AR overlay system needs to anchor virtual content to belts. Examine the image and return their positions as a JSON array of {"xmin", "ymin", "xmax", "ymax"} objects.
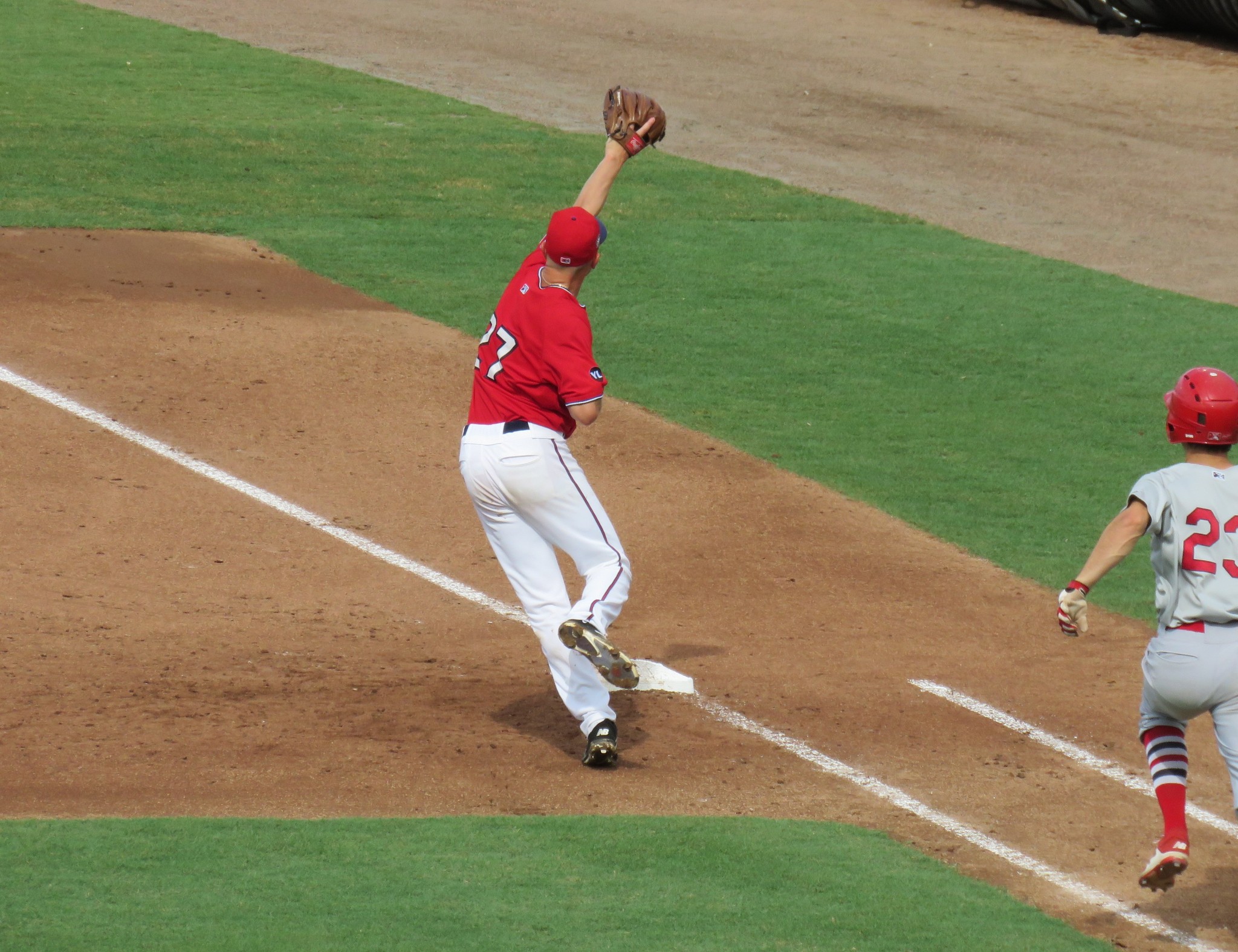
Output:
[
  {"xmin": 462, "ymin": 419, "xmax": 530, "ymax": 436},
  {"xmin": 1165, "ymin": 620, "xmax": 1205, "ymax": 633}
]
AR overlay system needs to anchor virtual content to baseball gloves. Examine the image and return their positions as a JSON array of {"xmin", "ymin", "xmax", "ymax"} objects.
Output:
[{"xmin": 601, "ymin": 83, "xmax": 669, "ymax": 159}]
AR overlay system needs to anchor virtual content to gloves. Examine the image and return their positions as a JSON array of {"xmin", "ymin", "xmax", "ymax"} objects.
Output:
[{"xmin": 1058, "ymin": 579, "xmax": 1091, "ymax": 639}]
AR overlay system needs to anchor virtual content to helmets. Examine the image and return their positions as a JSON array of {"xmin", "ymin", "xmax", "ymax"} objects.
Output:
[{"xmin": 1166, "ymin": 366, "xmax": 1238, "ymax": 445}]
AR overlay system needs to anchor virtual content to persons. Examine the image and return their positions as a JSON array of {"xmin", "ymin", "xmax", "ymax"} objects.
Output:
[
  {"xmin": 458, "ymin": 85, "xmax": 666, "ymax": 769},
  {"xmin": 1057, "ymin": 366, "xmax": 1238, "ymax": 894}
]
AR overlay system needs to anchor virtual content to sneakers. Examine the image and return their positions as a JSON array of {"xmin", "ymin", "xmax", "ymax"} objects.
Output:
[
  {"xmin": 558, "ymin": 617, "xmax": 639, "ymax": 689},
  {"xmin": 1138, "ymin": 833, "xmax": 1190, "ymax": 892},
  {"xmin": 580, "ymin": 718, "xmax": 618, "ymax": 768}
]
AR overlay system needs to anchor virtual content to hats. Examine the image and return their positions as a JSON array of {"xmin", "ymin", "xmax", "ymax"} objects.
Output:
[{"xmin": 547, "ymin": 206, "xmax": 607, "ymax": 267}]
